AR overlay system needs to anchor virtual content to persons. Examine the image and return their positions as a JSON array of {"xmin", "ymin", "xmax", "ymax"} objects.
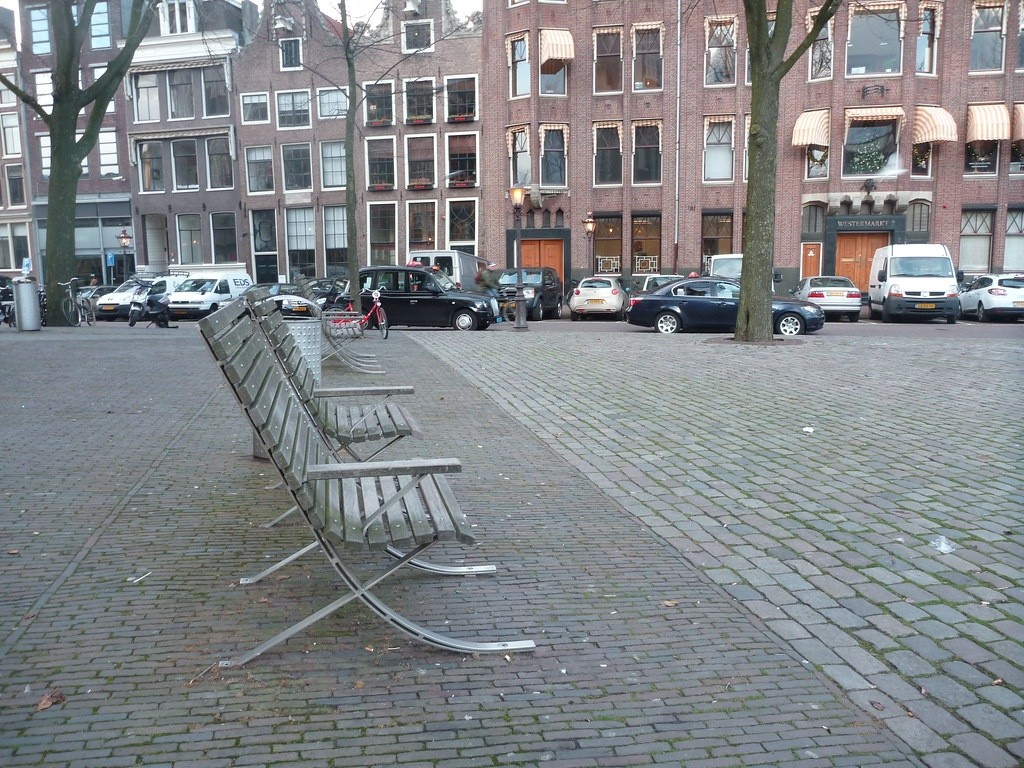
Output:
[{"xmin": 477, "ymin": 262, "xmax": 503, "ymax": 324}]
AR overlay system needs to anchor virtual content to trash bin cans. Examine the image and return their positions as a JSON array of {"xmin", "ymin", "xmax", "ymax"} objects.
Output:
[{"xmin": 12, "ymin": 275, "xmax": 43, "ymax": 330}]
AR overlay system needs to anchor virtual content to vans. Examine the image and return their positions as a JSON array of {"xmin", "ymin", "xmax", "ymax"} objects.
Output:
[
  {"xmin": 702, "ymin": 254, "xmax": 782, "ymax": 295},
  {"xmin": 867, "ymin": 244, "xmax": 963, "ymax": 324},
  {"xmin": 166, "ymin": 273, "xmax": 254, "ymax": 322},
  {"xmin": 407, "ymin": 250, "xmax": 494, "ymax": 293}
]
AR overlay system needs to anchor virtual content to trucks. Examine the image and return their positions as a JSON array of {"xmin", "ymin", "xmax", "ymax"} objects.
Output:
[{"xmin": 96, "ymin": 264, "xmax": 246, "ymax": 322}]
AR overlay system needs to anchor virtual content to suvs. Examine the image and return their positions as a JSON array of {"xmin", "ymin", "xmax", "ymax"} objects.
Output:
[{"xmin": 494, "ymin": 267, "xmax": 563, "ymax": 322}]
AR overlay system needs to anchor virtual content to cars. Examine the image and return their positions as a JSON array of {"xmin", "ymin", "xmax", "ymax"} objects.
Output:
[
  {"xmin": 217, "ymin": 279, "xmax": 342, "ymax": 316},
  {"xmin": 789, "ymin": 276, "xmax": 862, "ymax": 323},
  {"xmin": 335, "ymin": 262, "xmax": 492, "ymax": 333},
  {"xmin": 568, "ymin": 271, "xmax": 825, "ymax": 336},
  {"xmin": 957, "ymin": 274, "xmax": 1024, "ymax": 323},
  {"xmin": 78, "ymin": 285, "xmax": 118, "ymax": 314}
]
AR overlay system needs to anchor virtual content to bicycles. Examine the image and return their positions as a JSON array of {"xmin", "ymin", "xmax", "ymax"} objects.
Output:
[
  {"xmin": 0, "ymin": 286, "xmax": 47, "ymax": 328},
  {"xmin": 322, "ymin": 288, "xmax": 389, "ymax": 340},
  {"xmin": 57, "ymin": 278, "xmax": 96, "ymax": 327},
  {"xmin": 492, "ymin": 286, "xmax": 527, "ymax": 323}
]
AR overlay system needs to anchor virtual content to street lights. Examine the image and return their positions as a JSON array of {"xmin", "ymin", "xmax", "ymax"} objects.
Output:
[
  {"xmin": 581, "ymin": 212, "xmax": 597, "ymax": 277},
  {"xmin": 507, "ymin": 182, "xmax": 530, "ymax": 330},
  {"xmin": 115, "ymin": 229, "xmax": 133, "ymax": 282}
]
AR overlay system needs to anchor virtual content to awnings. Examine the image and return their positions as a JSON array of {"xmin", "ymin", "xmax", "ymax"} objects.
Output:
[
  {"xmin": 1013, "ymin": 104, "xmax": 1024, "ymax": 142},
  {"xmin": 592, "ymin": 25, "xmax": 624, "ymax": 61},
  {"xmin": 128, "ymin": 127, "xmax": 237, "ymax": 165},
  {"xmin": 805, "ymin": 6, "xmax": 835, "ymax": 41},
  {"xmin": 503, "ymin": 32, "xmax": 530, "ymax": 68},
  {"xmin": 540, "ymin": 29, "xmax": 575, "ymax": 67},
  {"xmin": 592, "ymin": 118, "xmax": 662, "ymax": 155},
  {"xmin": 701, "ymin": 114, "xmax": 753, "ymax": 150},
  {"xmin": 847, "ymin": 1, "xmax": 908, "ymax": 38},
  {"xmin": 918, "ymin": 1, "xmax": 943, "ymax": 39},
  {"xmin": 703, "ymin": 14, "xmax": 739, "ymax": 53},
  {"xmin": 913, "ymin": 104, "xmax": 959, "ymax": 145},
  {"xmin": 629, "ymin": 20, "xmax": 664, "ymax": 59},
  {"xmin": 505, "ymin": 124, "xmax": 571, "ymax": 158},
  {"xmin": 970, "ymin": 0, "xmax": 1011, "ymax": 39},
  {"xmin": 966, "ymin": 104, "xmax": 1011, "ymax": 144},
  {"xmin": 845, "ymin": 106, "xmax": 906, "ymax": 143},
  {"xmin": 125, "ymin": 57, "xmax": 231, "ymax": 98},
  {"xmin": 791, "ymin": 110, "xmax": 829, "ymax": 147}
]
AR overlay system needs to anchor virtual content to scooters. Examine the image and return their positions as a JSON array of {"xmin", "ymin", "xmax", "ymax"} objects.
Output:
[{"xmin": 128, "ymin": 275, "xmax": 170, "ymax": 328}]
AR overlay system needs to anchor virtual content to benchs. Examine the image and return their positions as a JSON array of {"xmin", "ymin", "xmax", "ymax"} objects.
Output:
[{"xmin": 198, "ymin": 279, "xmax": 537, "ymax": 668}]
